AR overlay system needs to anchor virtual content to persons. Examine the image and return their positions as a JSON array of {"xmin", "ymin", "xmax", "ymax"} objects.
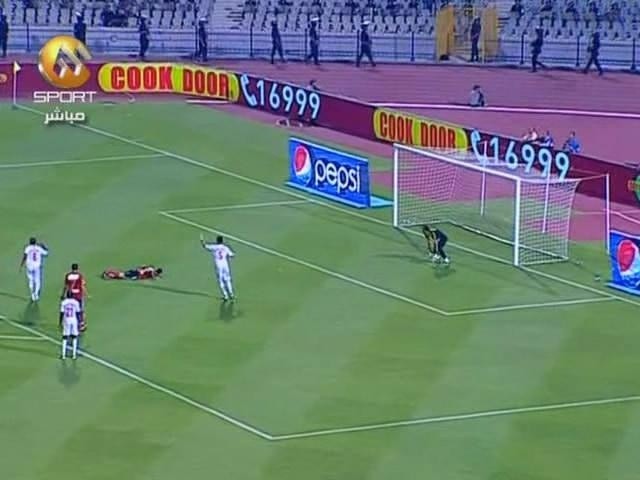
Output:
[
  {"xmin": 468, "ymin": 17, "xmax": 481, "ymax": 62},
  {"xmin": 270, "ymin": 21, "xmax": 287, "ymax": 64},
  {"xmin": 302, "ymin": 18, "xmax": 322, "ymax": 65},
  {"xmin": 580, "ymin": 32, "xmax": 604, "ymax": 76},
  {"xmin": 0, "ymin": 10, "xmax": 9, "ymax": 58},
  {"xmin": 528, "ymin": 28, "xmax": 547, "ymax": 73},
  {"xmin": 18, "ymin": 237, "xmax": 88, "ymax": 360},
  {"xmin": 196, "ymin": 18, "xmax": 210, "ymax": 63},
  {"xmin": 522, "ymin": 126, "xmax": 581, "ymax": 154},
  {"xmin": 72, "ymin": 11, "xmax": 87, "ymax": 58},
  {"xmin": 307, "ymin": 79, "xmax": 320, "ymax": 91},
  {"xmin": 138, "ymin": 16, "xmax": 150, "ymax": 61},
  {"xmin": 199, "ymin": 232, "xmax": 235, "ymax": 301},
  {"xmin": 422, "ymin": 223, "xmax": 449, "ymax": 264},
  {"xmin": 469, "ymin": 84, "xmax": 485, "ymax": 107},
  {"xmin": 509, "ymin": 1, "xmax": 623, "ymax": 14},
  {"xmin": 358, "ymin": 21, "xmax": 377, "ymax": 67},
  {"xmin": 102, "ymin": 263, "xmax": 163, "ymax": 280}
]
